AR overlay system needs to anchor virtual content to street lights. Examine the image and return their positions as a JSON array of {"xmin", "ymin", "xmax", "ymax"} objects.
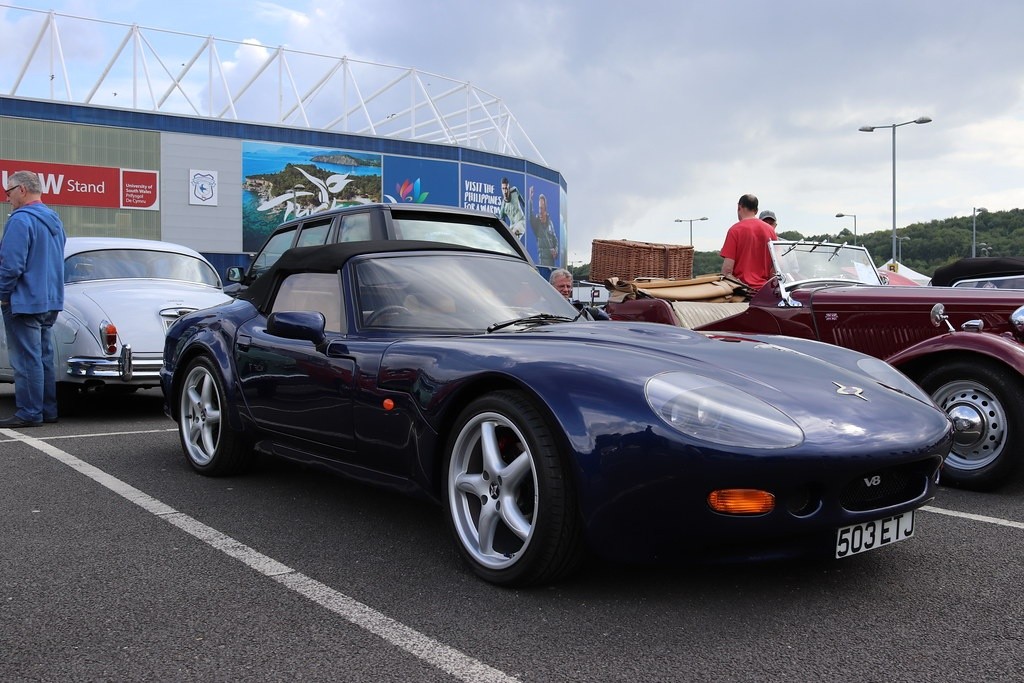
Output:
[
  {"xmin": 568, "ymin": 261, "xmax": 582, "ymax": 274},
  {"xmin": 858, "ymin": 116, "xmax": 932, "ymax": 265},
  {"xmin": 890, "ymin": 235, "xmax": 910, "ymax": 264},
  {"xmin": 835, "ymin": 213, "xmax": 856, "ymax": 245},
  {"xmin": 972, "ymin": 207, "xmax": 988, "ymax": 258},
  {"xmin": 675, "ymin": 217, "xmax": 708, "ymax": 245}
]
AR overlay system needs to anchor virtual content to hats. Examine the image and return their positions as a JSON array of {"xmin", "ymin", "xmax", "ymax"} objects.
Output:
[{"xmin": 759, "ymin": 210, "xmax": 777, "ymax": 222}]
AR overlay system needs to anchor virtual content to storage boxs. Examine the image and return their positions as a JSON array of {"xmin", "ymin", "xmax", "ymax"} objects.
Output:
[{"xmin": 573, "ymin": 286, "xmax": 609, "ymax": 303}]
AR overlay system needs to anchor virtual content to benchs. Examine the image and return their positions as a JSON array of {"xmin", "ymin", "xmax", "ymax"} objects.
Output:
[{"xmin": 671, "ymin": 301, "xmax": 749, "ymax": 329}]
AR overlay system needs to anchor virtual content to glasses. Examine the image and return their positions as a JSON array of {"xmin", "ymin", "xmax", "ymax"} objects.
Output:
[{"xmin": 5, "ymin": 184, "xmax": 21, "ymax": 198}]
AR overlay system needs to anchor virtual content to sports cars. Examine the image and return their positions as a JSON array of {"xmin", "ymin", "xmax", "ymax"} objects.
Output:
[
  {"xmin": 158, "ymin": 240, "xmax": 955, "ymax": 587},
  {"xmin": 607, "ymin": 239, "xmax": 1024, "ymax": 490},
  {"xmin": 932, "ymin": 258, "xmax": 1024, "ymax": 291}
]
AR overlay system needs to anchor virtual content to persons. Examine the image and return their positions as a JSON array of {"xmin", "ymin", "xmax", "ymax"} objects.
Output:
[
  {"xmin": 0, "ymin": 170, "xmax": 66, "ymax": 428},
  {"xmin": 550, "ymin": 269, "xmax": 573, "ymax": 300},
  {"xmin": 720, "ymin": 193, "xmax": 777, "ymax": 292},
  {"xmin": 528, "ymin": 187, "xmax": 559, "ymax": 267},
  {"xmin": 498, "ymin": 178, "xmax": 526, "ymax": 240},
  {"xmin": 759, "ymin": 210, "xmax": 799, "ymax": 274}
]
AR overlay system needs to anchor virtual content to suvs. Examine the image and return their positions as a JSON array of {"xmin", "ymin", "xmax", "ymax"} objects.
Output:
[{"xmin": 224, "ymin": 203, "xmax": 540, "ymax": 300}]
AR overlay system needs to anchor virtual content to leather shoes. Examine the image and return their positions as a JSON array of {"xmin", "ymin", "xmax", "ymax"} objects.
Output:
[
  {"xmin": 44, "ymin": 418, "xmax": 58, "ymax": 423},
  {"xmin": 0, "ymin": 414, "xmax": 43, "ymax": 428}
]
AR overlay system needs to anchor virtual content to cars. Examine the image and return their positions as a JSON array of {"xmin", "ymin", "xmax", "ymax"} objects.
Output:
[{"xmin": 0, "ymin": 237, "xmax": 234, "ymax": 413}]
